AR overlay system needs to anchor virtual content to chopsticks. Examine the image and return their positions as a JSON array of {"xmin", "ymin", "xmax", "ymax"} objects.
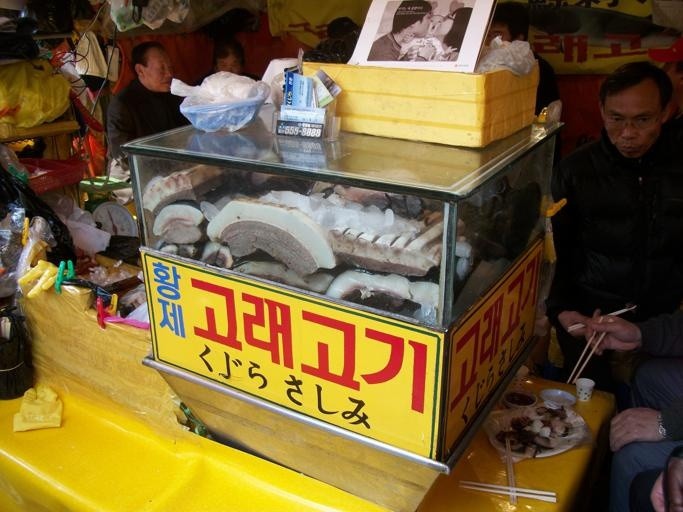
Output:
[
  {"xmin": 458, "ymin": 480, "xmax": 557, "ymax": 504},
  {"xmin": 567, "ymin": 306, "xmax": 637, "ymax": 332},
  {"xmin": 505, "ymin": 439, "xmax": 517, "ymax": 506},
  {"xmin": 567, "ymin": 316, "xmax": 614, "ymax": 384}
]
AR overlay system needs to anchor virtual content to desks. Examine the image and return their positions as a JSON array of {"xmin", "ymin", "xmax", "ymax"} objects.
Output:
[{"xmin": 0, "ymin": 256, "xmax": 614, "ymax": 512}]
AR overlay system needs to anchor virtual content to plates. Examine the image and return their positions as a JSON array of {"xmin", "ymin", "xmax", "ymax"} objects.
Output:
[
  {"xmin": 539, "ymin": 389, "xmax": 576, "ymax": 406},
  {"xmin": 503, "ymin": 390, "xmax": 537, "ymax": 408},
  {"xmin": 489, "ymin": 404, "xmax": 586, "ymax": 458}
]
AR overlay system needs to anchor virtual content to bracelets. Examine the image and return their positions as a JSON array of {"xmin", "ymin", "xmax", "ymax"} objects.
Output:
[{"xmin": 657, "ymin": 412, "xmax": 666, "ymax": 440}]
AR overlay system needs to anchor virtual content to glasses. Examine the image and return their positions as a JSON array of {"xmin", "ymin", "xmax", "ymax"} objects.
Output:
[{"xmin": 602, "ymin": 108, "xmax": 664, "ymax": 130}]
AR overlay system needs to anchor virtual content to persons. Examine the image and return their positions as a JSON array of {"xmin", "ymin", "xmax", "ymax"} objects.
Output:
[
  {"xmin": 552, "ymin": 61, "xmax": 681, "ymax": 411},
  {"xmin": 432, "ymin": 7, "xmax": 473, "ymax": 62},
  {"xmin": 103, "ymin": 41, "xmax": 192, "ymax": 201},
  {"xmin": 399, "ymin": 15, "xmax": 457, "ymax": 62},
  {"xmin": 367, "ymin": 0, "xmax": 433, "ymax": 61},
  {"xmin": 187, "ymin": 38, "xmax": 259, "ymax": 89},
  {"xmin": 586, "ymin": 304, "xmax": 683, "ymax": 511},
  {"xmin": 629, "ymin": 444, "xmax": 682, "ymax": 512},
  {"xmin": 300, "ymin": 16, "xmax": 362, "ymax": 64}
]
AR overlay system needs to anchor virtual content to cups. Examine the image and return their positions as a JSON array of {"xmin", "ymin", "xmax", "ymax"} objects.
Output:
[{"xmin": 575, "ymin": 378, "xmax": 595, "ymax": 401}]
